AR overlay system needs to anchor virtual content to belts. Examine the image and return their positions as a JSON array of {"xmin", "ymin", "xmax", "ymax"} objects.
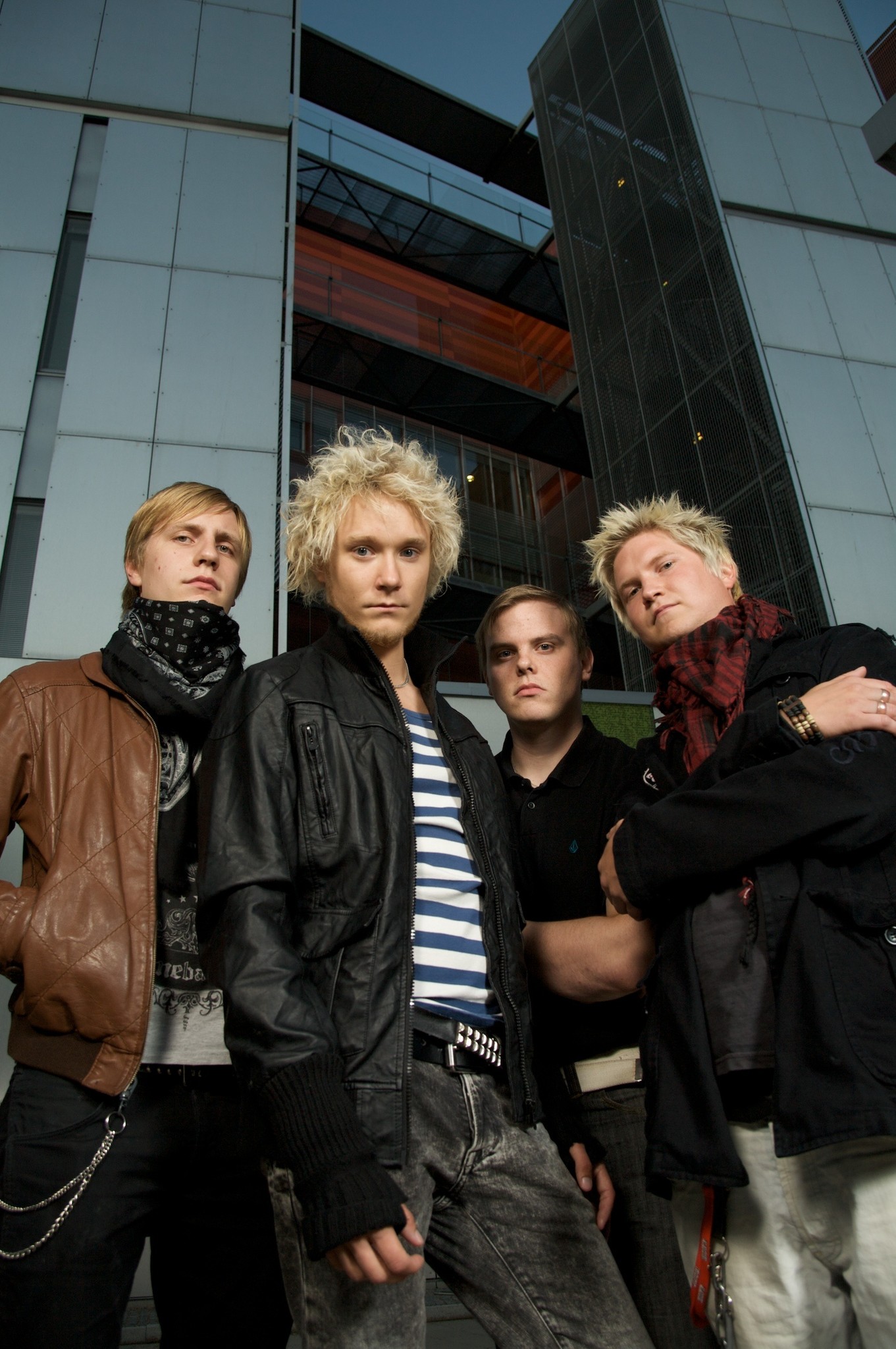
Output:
[
  {"xmin": 575, "ymin": 1048, "xmax": 644, "ymax": 1093},
  {"xmin": 407, "ymin": 1033, "xmax": 486, "ymax": 1074},
  {"xmin": 405, "ymin": 1014, "xmax": 503, "ymax": 1068}
]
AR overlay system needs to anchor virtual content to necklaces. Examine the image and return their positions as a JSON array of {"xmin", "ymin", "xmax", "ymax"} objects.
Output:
[{"xmin": 393, "ymin": 658, "xmax": 410, "ymax": 688}]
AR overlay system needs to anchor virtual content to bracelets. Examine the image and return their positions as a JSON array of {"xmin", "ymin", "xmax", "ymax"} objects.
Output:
[{"xmin": 776, "ymin": 694, "xmax": 826, "ymax": 746}]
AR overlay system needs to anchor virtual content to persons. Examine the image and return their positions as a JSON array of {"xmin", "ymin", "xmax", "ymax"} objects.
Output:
[
  {"xmin": 0, "ymin": 483, "xmax": 294, "ymax": 1349},
  {"xmin": 196, "ymin": 423, "xmax": 656, "ymax": 1349},
  {"xmin": 584, "ymin": 491, "xmax": 896, "ymax": 1349},
  {"xmin": 476, "ymin": 585, "xmax": 724, "ymax": 1349}
]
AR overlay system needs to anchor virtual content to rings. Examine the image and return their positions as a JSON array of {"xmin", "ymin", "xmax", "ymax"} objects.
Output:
[
  {"xmin": 880, "ymin": 689, "xmax": 890, "ymax": 703},
  {"xmin": 875, "ymin": 702, "xmax": 887, "ymax": 716}
]
